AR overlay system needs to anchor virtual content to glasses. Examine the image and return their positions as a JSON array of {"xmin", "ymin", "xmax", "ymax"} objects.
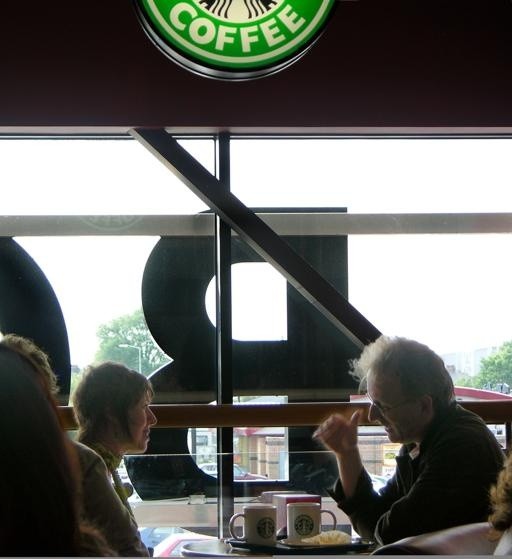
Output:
[{"xmin": 366, "ymin": 392, "xmax": 420, "ymax": 415}]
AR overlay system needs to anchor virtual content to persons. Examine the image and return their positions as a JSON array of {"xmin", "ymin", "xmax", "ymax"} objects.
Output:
[
  {"xmin": 68, "ymin": 357, "xmax": 159, "ymax": 556},
  {"xmin": 1, "ymin": 331, "xmax": 123, "ymax": 557},
  {"xmin": 310, "ymin": 332, "xmax": 511, "ymax": 546},
  {"xmin": 481, "ymin": 439, "xmax": 512, "ymax": 558}
]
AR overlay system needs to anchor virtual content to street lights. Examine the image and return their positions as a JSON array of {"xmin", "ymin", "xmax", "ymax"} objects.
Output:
[{"xmin": 118, "ymin": 343, "xmax": 142, "ymax": 374}]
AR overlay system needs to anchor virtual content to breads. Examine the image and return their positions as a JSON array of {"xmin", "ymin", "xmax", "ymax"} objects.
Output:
[{"xmin": 311, "ymin": 531, "xmax": 350, "ymax": 544}]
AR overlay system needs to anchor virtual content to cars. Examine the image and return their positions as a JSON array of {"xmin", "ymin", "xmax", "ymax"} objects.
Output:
[{"xmin": 200, "ymin": 462, "xmax": 269, "ymax": 480}]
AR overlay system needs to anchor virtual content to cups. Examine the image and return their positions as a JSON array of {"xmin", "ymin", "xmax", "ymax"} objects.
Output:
[
  {"xmin": 286, "ymin": 503, "xmax": 338, "ymax": 538},
  {"xmin": 228, "ymin": 507, "xmax": 279, "ymax": 547}
]
